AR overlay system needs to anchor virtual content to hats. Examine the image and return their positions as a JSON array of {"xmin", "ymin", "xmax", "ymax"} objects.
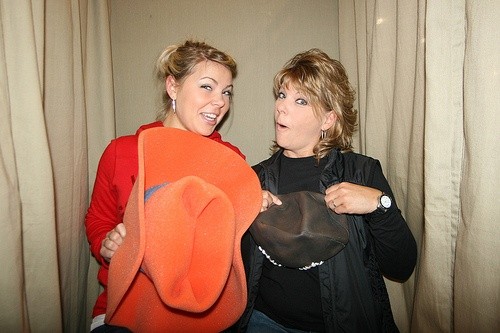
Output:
[
  {"xmin": 105, "ymin": 127, "xmax": 262, "ymax": 333},
  {"xmin": 250, "ymin": 191, "xmax": 350, "ymax": 269}
]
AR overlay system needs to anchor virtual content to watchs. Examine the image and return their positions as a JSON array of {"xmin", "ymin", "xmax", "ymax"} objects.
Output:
[{"xmin": 373, "ymin": 185, "xmax": 394, "ymax": 217}]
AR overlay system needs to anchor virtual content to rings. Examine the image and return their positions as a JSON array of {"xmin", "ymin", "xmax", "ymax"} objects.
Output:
[{"xmin": 332, "ymin": 199, "xmax": 337, "ymax": 208}]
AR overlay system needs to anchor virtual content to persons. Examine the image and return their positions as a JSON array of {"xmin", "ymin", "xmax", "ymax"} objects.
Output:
[
  {"xmin": 85, "ymin": 42, "xmax": 262, "ymax": 333},
  {"xmin": 237, "ymin": 49, "xmax": 418, "ymax": 333}
]
